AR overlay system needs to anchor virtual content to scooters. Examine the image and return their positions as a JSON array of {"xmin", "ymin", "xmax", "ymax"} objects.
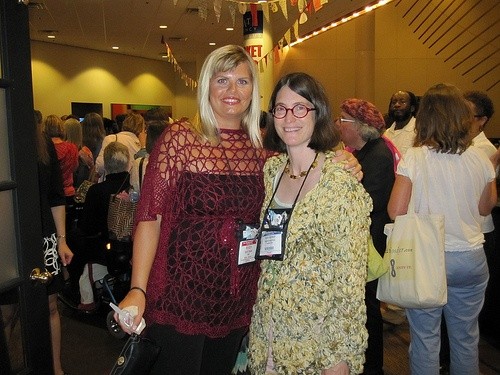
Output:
[{"xmin": 58, "ymin": 240, "xmax": 134, "ymax": 340}]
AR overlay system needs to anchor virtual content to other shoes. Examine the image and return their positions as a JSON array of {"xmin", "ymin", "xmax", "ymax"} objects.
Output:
[{"xmin": 79, "ymin": 301, "xmax": 96, "ymax": 311}]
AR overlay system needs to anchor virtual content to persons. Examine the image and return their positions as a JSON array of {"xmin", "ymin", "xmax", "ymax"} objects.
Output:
[
  {"xmin": 45, "ymin": 116, "xmax": 78, "ymax": 288},
  {"xmin": 335, "ymin": 98, "xmax": 395, "ymax": 374},
  {"xmin": 61, "ymin": 142, "xmax": 130, "ymax": 310},
  {"xmin": 247, "ymin": 72, "xmax": 374, "ymax": 374},
  {"xmin": 112, "ymin": 44, "xmax": 364, "ymax": 375},
  {"xmin": 37, "ymin": 127, "xmax": 74, "ymax": 375},
  {"xmin": 462, "ymin": 89, "xmax": 499, "ymax": 346},
  {"xmin": 129, "ymin": 121, "xmax": 173, "ymax": 199},
  {"xmin": 387, "ymin": 83, "xmax": 497, "ymax": 374},
  {"xmin": 380, "ymin": 88, "xmax": 416, "ymax": 162},
  {"xmin": 62, "ymin": 118, "xmax": 94, "ymax": 200},
  {"xmin": 33, "ymin": 107, "xmax": 179, "ymax": 182}
]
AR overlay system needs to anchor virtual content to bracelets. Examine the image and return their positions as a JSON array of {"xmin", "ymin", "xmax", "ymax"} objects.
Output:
[
  {"xmin": 55, "ymin": 233, "xmax": 66, "ymax": 238},
  {"xmin": 129, "ymin": 286, "xmax": 146, "ymax": 299}
]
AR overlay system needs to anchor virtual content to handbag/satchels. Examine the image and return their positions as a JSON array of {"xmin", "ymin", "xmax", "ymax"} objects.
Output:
[
  {"xmin": 109, "ymin": 287, "xmax": 152, "ymax": 375},
  {"xmin": 75, "ymin": 165, "xmax": 99, "ymax": 204},
  {"xmin": 107, "ymin": 163, "xmax": 138, "ymax": 242},
  {"xmin": 377, "ymin": 149, "xmax": 447, "ymax": 310}
]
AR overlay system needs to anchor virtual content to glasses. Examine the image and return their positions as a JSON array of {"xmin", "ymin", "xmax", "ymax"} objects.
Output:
[
  {"xmin": 338, "ymin": 116, "xmax": 356, "ymax": 124},
  {"xmin": 272, "ymin": 104, "xmax": 316, "ymax": 118}
]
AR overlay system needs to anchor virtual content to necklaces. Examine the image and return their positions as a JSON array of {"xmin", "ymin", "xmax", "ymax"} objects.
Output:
[{"xmin": 284, "ymin": 151, "xmax": 321, "ymax": 180}]
